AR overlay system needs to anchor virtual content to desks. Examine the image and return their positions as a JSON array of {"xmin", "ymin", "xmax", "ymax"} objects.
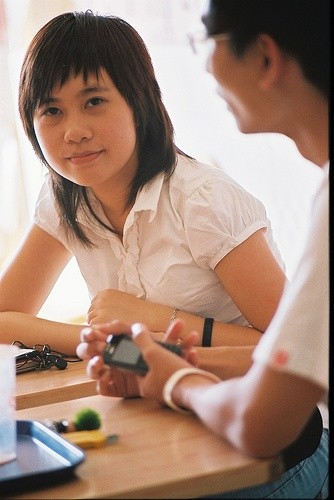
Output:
[{"xmin": 0, "ymin": 354, "xmax": 324, "ymax": 500}]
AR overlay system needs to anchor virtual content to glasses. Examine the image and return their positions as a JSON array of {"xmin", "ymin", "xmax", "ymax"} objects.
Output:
[{"xmin": 186, "ymin": 28, "xmax": 231, "ymax": 54}]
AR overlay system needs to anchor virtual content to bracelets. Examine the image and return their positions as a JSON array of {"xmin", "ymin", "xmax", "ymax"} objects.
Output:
[
  {"xmin": 167, "ymin": 306, "xmax": 182, "ymax": 345},
  {"xmin": 201, "ymin": 318, "xmax": 214, "ymax": 347},
  {"xmin": 163, "ymin": 366, "xmax": 223, "ymax": 415}
]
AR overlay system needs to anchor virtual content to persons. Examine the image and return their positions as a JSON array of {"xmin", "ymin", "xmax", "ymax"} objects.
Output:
[
  {"xmin": 76, "ymin": 0, "xmax": 330, "ymax": 459},
  {"xmin": 0, "ymin": 9, "xmax": 328, "ymax": 499}
]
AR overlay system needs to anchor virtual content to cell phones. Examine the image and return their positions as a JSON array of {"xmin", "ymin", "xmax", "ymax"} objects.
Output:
[{"xmin": 103, "ymin": 334, "xmax": 182, "ymax": 374}]
